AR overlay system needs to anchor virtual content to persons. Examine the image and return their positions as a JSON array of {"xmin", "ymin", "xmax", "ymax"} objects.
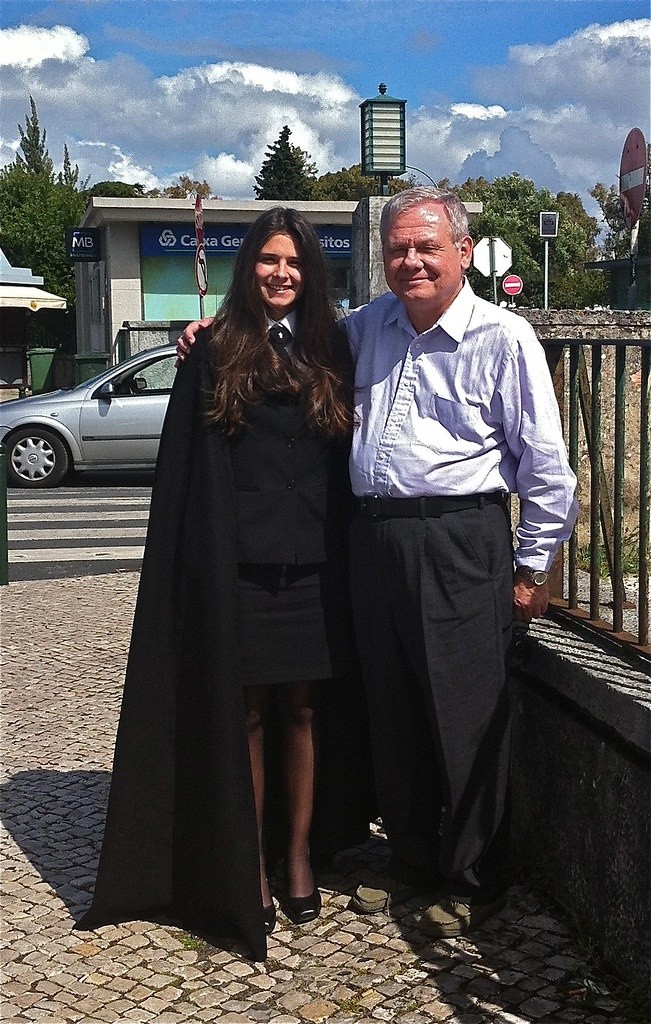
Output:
[
  {"xmin": 72, "ymin": 206, "xmax": 355, "ymax": 962},
  {"xmin": 174, "ymin": 186, "xmax": 579, "ymax": 939}
]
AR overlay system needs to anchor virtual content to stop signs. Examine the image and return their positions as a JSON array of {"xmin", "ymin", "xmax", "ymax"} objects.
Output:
[
  {"xmin": 619, "ymin": 126, "xmax": 647, "ymax": 232},
  {"xmin": 502, "ymin": 275, "xmax": 523, "ymax": 295}
]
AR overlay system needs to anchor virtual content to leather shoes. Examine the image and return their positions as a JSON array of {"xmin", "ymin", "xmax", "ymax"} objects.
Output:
[
  {"xmin": 284, "ymin": 887, "xmax": 321, "ymax": 924},
  {"xmin": 263, "ymin": 904, "xmax": 276, "ymax": 933}
]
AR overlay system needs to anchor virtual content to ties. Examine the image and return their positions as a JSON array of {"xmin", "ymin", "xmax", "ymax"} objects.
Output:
[{"xmin": 267, "ymin": 327, "xmax": 293, "ymax": 383}]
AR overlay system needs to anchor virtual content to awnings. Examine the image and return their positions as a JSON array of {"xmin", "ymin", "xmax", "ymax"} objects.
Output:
[{"xmin": 0, "ymin": 286, "xmax": 67, "ymax": 311}]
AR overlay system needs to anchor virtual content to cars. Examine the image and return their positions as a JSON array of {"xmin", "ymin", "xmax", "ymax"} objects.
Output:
[{"xmin": 0, "ymin": 344, "xmax": 180, "ymax": 486}]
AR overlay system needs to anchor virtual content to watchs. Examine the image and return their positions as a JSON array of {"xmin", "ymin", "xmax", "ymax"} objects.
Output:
[{"xmin": 515, "ymin": 564, "xmax": 549, "ymax": 586}]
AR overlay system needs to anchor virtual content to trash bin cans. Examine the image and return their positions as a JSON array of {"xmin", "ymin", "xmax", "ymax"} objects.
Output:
[
  {"xmin": 74, "ymin": 351, "xmax": 111, "ymax": 385},
  {"xmin": 26, "ymin": 348, "xmax": 59, "ymax": 395}
]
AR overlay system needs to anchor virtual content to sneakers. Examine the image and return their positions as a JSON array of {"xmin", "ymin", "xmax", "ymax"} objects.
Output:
[
  {"xmin": 354, "ymin": 843, "xmax": 440, "ymax": 913},
  {"xmin": 418, "ymin": 897, "xmax": 508, "ymax": 937}
]
